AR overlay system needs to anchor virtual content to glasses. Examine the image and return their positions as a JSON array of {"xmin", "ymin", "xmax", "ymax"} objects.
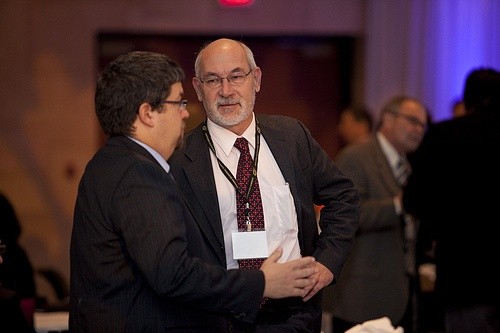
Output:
[
  {"xmin": 199, "ymin": 69, "xmax": 252, "ymax": 88},
  {"xmin": 160, "ymin": 100, "xmax": 188, "ymax": 111},
  {"xmin": 393, "ymin": 112, "xmax": 428, "ymax": 133}
]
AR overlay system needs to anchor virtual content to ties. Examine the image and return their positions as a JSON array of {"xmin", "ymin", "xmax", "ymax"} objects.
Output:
[
  {"xmin": 233, "ymin": 137, "xmax": 268, "ymax": 313},
  {"xmin": 394, "ymin": 158, "xmax": 418, "ymax": 280}
]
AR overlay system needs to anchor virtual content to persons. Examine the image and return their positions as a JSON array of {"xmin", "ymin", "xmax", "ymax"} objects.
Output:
[
  {"xmin": 69, "ymin": 51, "xmax": 319, "ymax": 333},
  {"xmin": 334, "ymin": 102, "xmax": 373, "ymax": 145},
  {"xmin": 167, "ymin": 38, "xmax": 362, "ymax": 333},
  {"xmin": 401, "ymin": 66, "xmax": 500, "ymax": 333},
  {"xmin": 319, "ymin": 96, "xmax": 426, "ymax": 333}
]
